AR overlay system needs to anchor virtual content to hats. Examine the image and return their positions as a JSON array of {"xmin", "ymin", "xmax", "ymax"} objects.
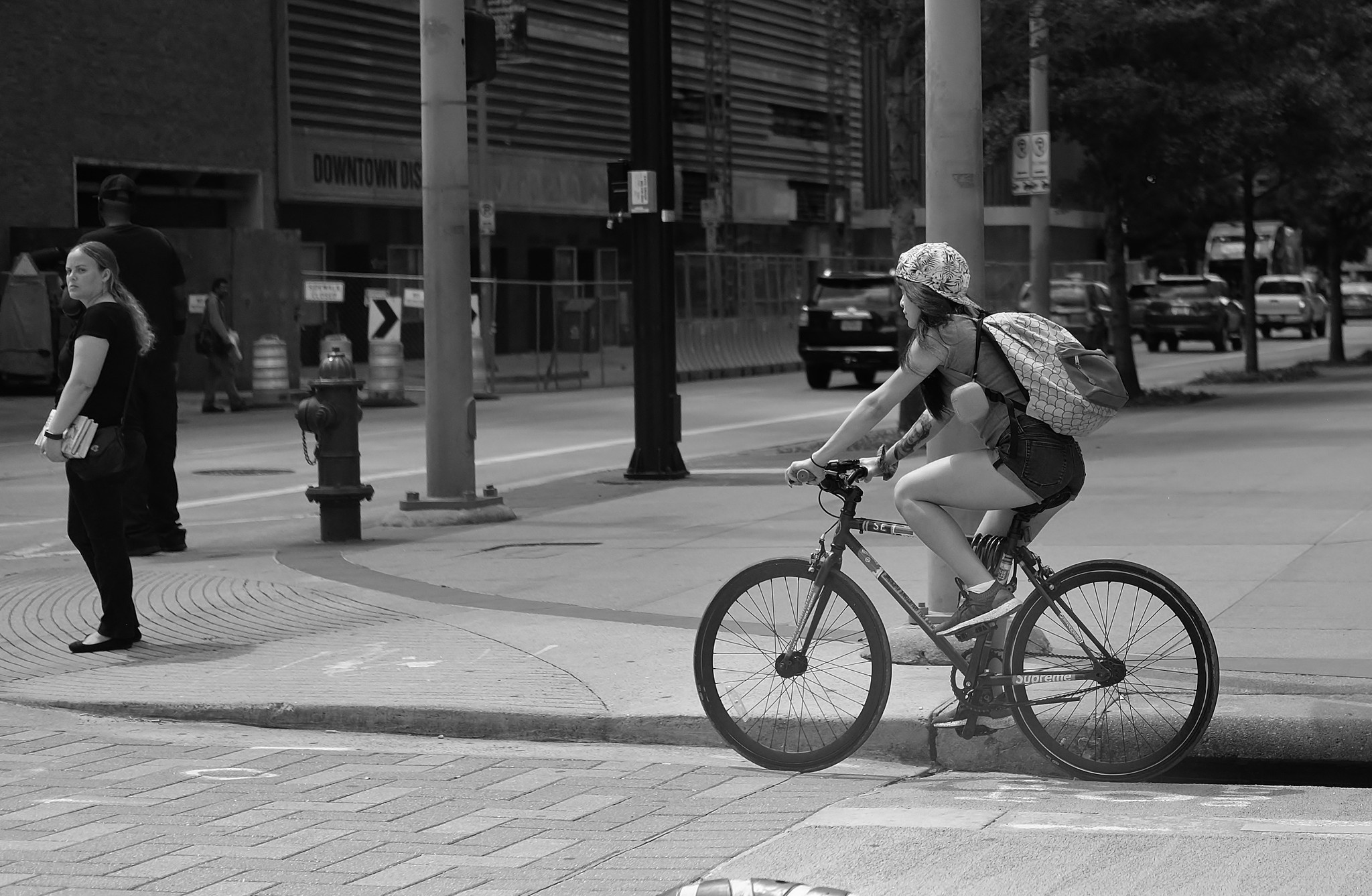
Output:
[
  {"xmin": 94, "ymin": 173, "xmax": 139, "ymax": 204},
  {"xmin": 895, "ymin": 241, "xmax": 979, "ymax": 308}
]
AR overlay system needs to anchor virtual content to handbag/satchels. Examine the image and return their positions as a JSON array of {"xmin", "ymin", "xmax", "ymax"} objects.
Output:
[
  {"xmin": 196, "ymin": 331, "xmax": 210, "ymax": 355},
  {"xmin": 72, "ymin": 425, "xmax": 126, "ymax": 482}
]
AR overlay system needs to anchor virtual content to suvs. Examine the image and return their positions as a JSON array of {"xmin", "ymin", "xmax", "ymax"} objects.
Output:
[{"xmin": 796, "ymin": 269, "xmax": 915, "ymax": 390}]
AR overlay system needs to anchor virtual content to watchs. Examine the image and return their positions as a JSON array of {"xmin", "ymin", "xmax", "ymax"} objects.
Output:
[{"xmin": 44, "ymin": 430, "xmax": 63, "ymax": 440}]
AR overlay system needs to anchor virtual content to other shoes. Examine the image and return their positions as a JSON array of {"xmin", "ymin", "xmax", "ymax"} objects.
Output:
[
  {"xmin": 203, "ymin": 404, "xmax": 225, "ymax": 414},
  {"xmin": 231, "ymin": 405, "xmax": 252, "ymax": 413},
  {"xmin": 126, "ymin": 542, "xmax": 160, "ymax": 557},
  {"xmin": 69, "ymin": 625, "xmax": 141, "ymax": 652},
  {"xmin": 160, "ymin": 533, "xmax": 187, "ymax": 552}
]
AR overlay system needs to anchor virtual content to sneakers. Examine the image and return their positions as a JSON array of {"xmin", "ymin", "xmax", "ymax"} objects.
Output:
[
  {"xmin": 941, "ymin": 577, "xmax": 1025, "ymax": 639},
  {"xmin": 933, "ymin": 690, "xmax": 1015, "ymax": 730}
]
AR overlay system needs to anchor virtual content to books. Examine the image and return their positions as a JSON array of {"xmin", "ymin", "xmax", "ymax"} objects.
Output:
[{"xmin": 34, "ymin": 409, "xmax": 99, "ymax": 459}]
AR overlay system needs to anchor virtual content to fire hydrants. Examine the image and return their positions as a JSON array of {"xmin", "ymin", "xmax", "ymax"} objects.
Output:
[{"xmin": 294, "ymin": 346, "xmax": 375, "ymax": 542}]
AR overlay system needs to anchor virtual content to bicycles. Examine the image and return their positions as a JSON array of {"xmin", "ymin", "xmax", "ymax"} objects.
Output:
[{"xmin": 692, "ymin": 463, "xmax": 1221, "ymax": 785}]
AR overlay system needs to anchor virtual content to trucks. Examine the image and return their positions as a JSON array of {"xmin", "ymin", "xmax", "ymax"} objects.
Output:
[{"xmin": 1202, "ymin": 221, "xmax": 1304, "ymax": 302}]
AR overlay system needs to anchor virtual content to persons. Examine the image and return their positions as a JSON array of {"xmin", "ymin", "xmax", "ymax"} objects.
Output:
[
  {"xmin": 41, "ymin": 241, "xmax": 154, "ymax": 654},
  {"xmin": 785, "ymin": 242, "xmax": 1086, "ymax": 729},
  {"xmin": 58, "ymin": 174, "xmax": 188, "ymax": 558},
  {"xmin": 200, "ymin": 278, "xmax": 248, "ymax": 413}
]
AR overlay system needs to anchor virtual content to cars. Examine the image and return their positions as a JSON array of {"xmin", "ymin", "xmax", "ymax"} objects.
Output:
[
  {"xmin": 1340, "ymin": 280, "xmax": 1372, "ymax": 316},
  {"xmin": 1016, "ymin": 279, "xmax": 1114, "ymax": 351},
  {"xmin": 1126, "ymin": 280, "xmax": 1156, "ymax": 328},
  {"xmin": 1253, "ymin": 274, "xmax": 1328, "ymax": 340},
  {"xmin": 1142, "ymin": 272, "xmax": 1245, "ymax": 353}
]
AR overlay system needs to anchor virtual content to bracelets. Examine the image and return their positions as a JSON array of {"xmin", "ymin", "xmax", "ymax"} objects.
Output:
[
  {"xmin": 876, "ymin": 444, "xmax": 899, "ymax": 481},
  {"xmin": 810, "ymin": 456, "xmax": 825, "ymax": 468}
]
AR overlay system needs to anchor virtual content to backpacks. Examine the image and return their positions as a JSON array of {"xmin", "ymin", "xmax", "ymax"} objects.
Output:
[{"xmin": 945, "ymin": 312, "xmax": 1129, "ymax": 436}]
